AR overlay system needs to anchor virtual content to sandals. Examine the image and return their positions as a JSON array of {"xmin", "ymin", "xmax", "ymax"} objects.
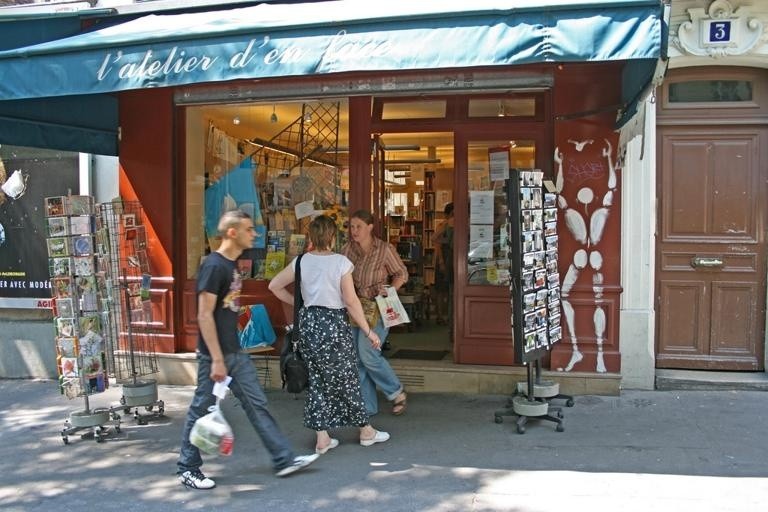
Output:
[{"xmin": 391, "ymin": 391, "xmax": 408, "ymax": 416}]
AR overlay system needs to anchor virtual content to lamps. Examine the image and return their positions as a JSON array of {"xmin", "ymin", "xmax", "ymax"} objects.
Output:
[
  {"xmin": 305, "ymin": 103, "xmax": 312, "ymax": 123},
  {"xmin": 496, "ymin": 100, "xmax": 504, "ymax": 117},
  {"xmin": 233, "ymin": 106, "xmax": 240, "ymax": 126},
  {"xmin": 510, "ymin": 140, "xmax": 518, "ymax": 149},
  {"xmin": 270, "ymin": 104, "xmax": 278, "ymax": 123}
]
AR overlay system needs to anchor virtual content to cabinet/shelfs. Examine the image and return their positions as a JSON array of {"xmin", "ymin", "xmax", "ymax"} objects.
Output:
[{"xmin": 383, "ymin": 164, "xmax": 453, "ymax": 334}]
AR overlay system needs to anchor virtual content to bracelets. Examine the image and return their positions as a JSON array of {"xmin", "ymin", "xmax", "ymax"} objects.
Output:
[{"xmin": 365, "ymin": 328, "xmax": 370, "ymax": 338}]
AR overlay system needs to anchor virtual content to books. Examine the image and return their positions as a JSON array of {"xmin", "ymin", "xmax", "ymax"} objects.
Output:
[
  {"xmin": 508, "ymin": 167, "xmax": 563, "ymax": 352},
  {"xmin": 45, "ymin": 196, "xmax": 152, "ymax": 400},
  {"xmin": 234, "ymin": 230, "xmax": 306, "ymax": 282}
]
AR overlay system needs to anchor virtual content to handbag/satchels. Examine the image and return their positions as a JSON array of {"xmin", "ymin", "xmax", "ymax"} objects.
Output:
[
  {"xmin": 346, "ymin": 298, "xmax": 380, "ymax": 328},
  {"xmin": 279, "ymin": 333, "xmax": 308, "ymax": 394},
  {"xmin": 375, "ymin": 285, "xmax": 411, "ymax": 330}
]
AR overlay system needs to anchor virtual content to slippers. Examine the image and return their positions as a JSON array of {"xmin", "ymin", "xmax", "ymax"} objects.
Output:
[
  {"xmin": 359, "ymin": 430, "xmax": 391, "ymax": 447},
  {"xmin": 316, "ymin": 438, "xmax": 339, "ymax": 455}
]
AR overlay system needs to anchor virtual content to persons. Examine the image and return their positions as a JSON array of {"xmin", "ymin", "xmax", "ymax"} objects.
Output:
[
  {"xmin": 177, "ymin": 209, "xmax": 319, "ymax": 488},
  {"xmin": 268, "ymin": 215, "xmax": 390, "ymax": 456},
  {"xmin": 340, "ymin": 210, "xmax": 409, "ymax": 417},
  {"xmin": 432, "ymin": 202, "xmax": 454, "ymax": 324}
]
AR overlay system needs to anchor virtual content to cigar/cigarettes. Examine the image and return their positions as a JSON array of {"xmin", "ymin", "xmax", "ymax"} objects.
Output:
[{"xmin": 378, "ymin": 346, "xmax": 382, "ymax": 352}]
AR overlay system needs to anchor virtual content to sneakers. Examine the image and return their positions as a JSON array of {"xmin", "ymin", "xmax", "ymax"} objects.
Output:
[
  {"xmin": 274, "ymin": 453, "xmax": 321, "ymax": 478},
  {"xmin": 177, "ymin": 469, "xmax": 217, "ymax": 489}
]
